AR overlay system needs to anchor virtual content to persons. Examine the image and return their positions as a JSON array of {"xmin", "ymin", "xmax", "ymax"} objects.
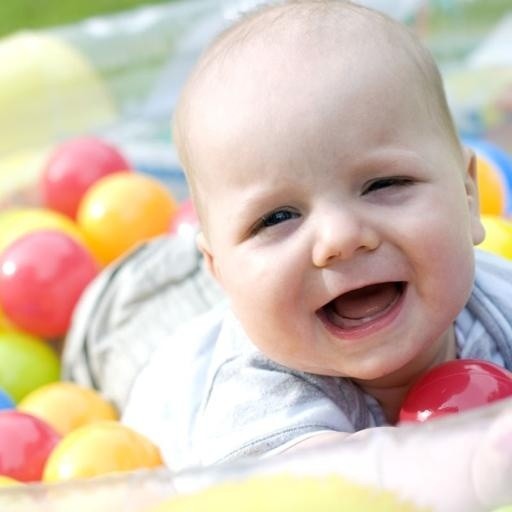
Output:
[{"xmin": 59, "ymin": 0, "xmax": 512, "ymax": 512}]
